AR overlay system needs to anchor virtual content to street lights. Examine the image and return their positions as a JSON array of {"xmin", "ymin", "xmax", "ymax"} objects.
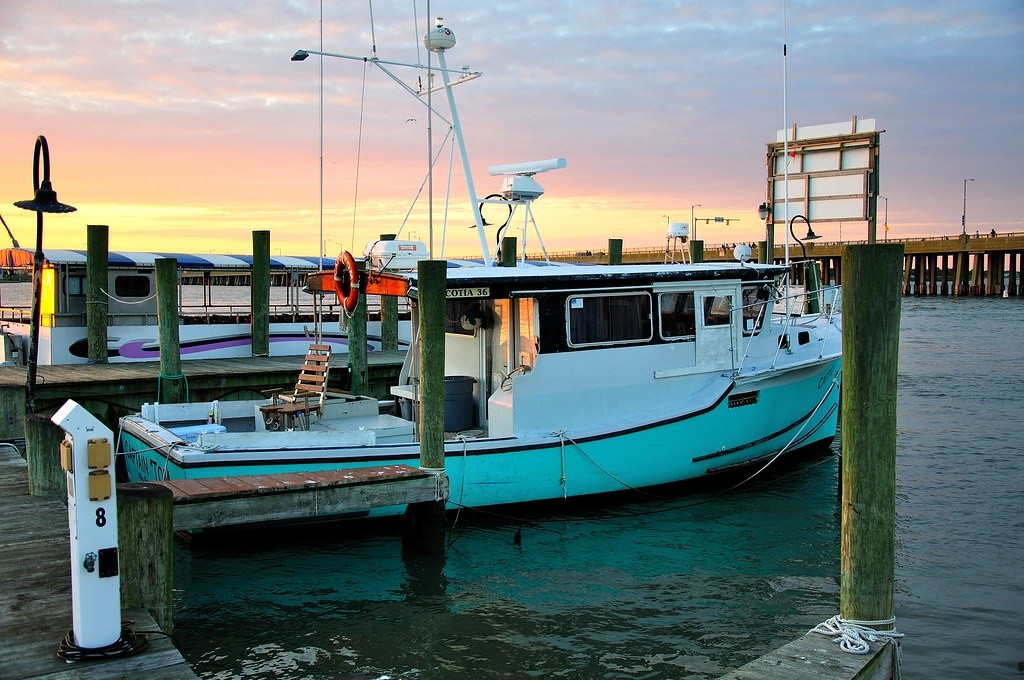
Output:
[
  {"xmin": 691, "ymin": 205, "xmax": 701, "ymax": 240},
  {"xmin": 961, "ymin": 178, "xmax": 975, "ymax": 234},
  {"xmin": 662, "ymin": 214, "xmax": 671, "ymax": 253},
  {"xmin": 877, "ymin": 196, "xmax": 888, "ymax": 243}
]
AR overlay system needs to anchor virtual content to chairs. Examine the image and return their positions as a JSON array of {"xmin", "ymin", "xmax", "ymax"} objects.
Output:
[{"xmin": 260, "ymin": 344, "xmax": 332, "ymax": 432}]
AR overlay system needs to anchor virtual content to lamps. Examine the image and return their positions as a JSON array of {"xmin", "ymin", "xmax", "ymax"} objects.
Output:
[
  {"xmin": 732, "ymin": 243, "xmax": 751, "ymax": 267},
  {"xmin": 757, "ymin": 202, "xmax": 770, "ymax": 219}
]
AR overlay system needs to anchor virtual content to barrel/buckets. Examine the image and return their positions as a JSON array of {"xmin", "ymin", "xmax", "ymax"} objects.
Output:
[{"xmin": 445, "ymin": 375, "xmax": 477, "ymax": 432}]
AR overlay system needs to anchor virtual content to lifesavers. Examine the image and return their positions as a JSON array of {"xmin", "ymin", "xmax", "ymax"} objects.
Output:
[{"xmin": 334, "ymin": 251, "xmax": 358, "ymax": 310}]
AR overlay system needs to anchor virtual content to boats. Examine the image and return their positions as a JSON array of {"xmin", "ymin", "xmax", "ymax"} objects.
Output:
[
  {"xmin": 118, "ymin": 17, "xmax": 842, "ymax": 531},
  {"xmin": 1, "ymin": 248, "xmax": 595, "ymax": 366}
]
